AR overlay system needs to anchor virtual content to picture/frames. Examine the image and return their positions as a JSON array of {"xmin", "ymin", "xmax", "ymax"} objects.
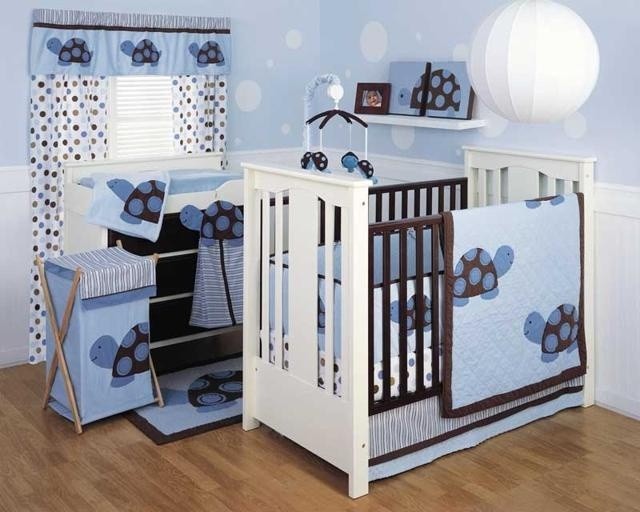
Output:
[{"xmin": 355, "ymin": 83, "xmax": 391, "ymax": 114}]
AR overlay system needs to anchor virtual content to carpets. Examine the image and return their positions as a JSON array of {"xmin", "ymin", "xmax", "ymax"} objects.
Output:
[{"xmin": 119, "ymin": 353, "xmax": 243, "ymax": 444}]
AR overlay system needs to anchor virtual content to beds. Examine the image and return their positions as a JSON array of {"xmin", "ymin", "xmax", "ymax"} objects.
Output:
[{"xmin": 243, "ymin": 145, "xmax": 595, "ymax": 496}]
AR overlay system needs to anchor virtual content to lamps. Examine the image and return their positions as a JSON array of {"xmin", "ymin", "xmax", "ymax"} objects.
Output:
[{"xmin": 466, "ymin": 0, "xmax": 601, "ymax": 124}]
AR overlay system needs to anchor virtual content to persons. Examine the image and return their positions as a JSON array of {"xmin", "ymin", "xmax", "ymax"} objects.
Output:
[{"xmin": 362, "ymin": 90, "xmax": 382, "ymax": 107}]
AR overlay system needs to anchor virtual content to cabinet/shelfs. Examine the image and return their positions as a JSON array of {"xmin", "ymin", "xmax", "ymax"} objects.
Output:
[{"xmin": 67, "ymin": 153, "xmax": 249, "ymax": 351}]
